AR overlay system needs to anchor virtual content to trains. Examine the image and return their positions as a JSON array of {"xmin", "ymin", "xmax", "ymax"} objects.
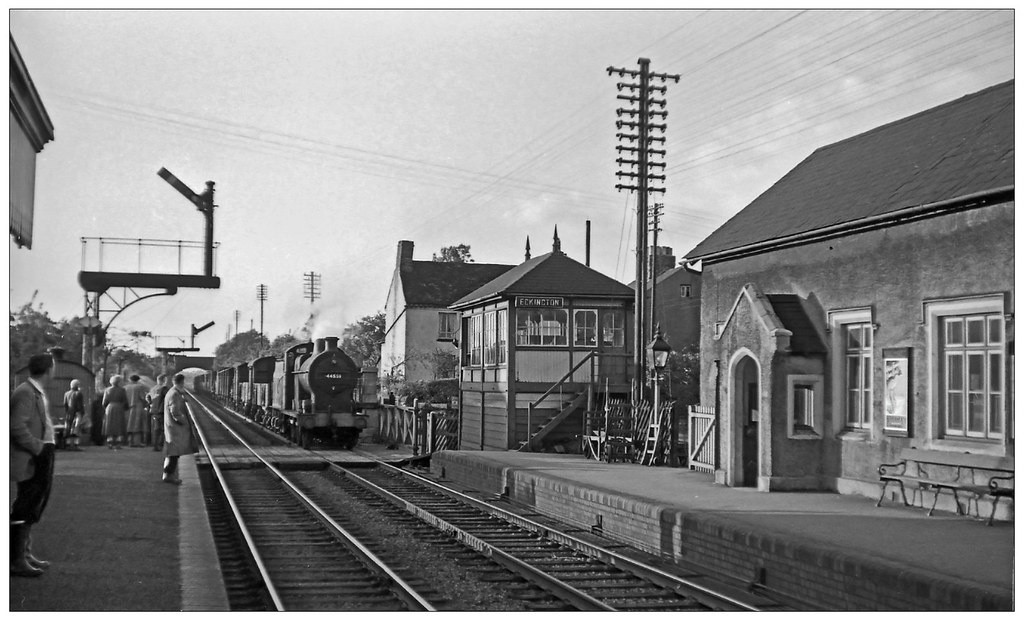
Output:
[{"xmin": 193, "ymin": 337, "xmax": 370, "ymax": 451}]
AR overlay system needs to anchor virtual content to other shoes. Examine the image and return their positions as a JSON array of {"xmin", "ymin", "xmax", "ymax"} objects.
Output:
[
  {"xmin": 129, "ymin": 443, "xmax": 133, "ymax": 447},
  {"xmin": 133, "ymin": 443, "xmax": 146, "ymax": 447},
  {"xmin": 74, "ymin": 445, "xmax": 84, "ymax": 451},
  {"xmin": 163, "ymin": 474, "xmax": 182, "ymax": 485},
  {"xmin": 65, "ymin": 445, "xmax": 73, "ymax": 451}
]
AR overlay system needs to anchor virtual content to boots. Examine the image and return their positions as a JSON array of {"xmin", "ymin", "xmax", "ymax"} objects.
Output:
[
  {"xmin": 9, "ymin": 522, "xmax": 43, "ymax": 577},
  {"xmin": 25, "ymin": 534, "xmax": 49, "ymax": 568}
]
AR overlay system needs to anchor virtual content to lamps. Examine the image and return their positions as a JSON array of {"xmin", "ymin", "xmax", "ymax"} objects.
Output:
[{"xmin": 645, "ymin": 317, "xmax": 672, "ymax": 377}]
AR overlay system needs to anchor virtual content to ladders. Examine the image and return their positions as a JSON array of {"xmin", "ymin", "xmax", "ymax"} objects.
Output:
[{"xmin": 639, "ymin": 408, "xmax": 665, "ymax": 466}]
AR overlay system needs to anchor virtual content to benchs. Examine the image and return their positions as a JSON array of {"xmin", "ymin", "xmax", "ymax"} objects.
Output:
[{"xmin": 866, "ymin": 447, "xmax": 1014, "ymax": 528}]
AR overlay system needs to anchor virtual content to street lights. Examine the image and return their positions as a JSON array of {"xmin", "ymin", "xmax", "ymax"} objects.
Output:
[{"xmin": 644, "ymin": 322, "xmax": 671, "ymax": 466}]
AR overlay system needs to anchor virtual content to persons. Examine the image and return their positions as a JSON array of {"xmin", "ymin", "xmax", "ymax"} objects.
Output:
[
  {"xmin": 9, "ymin": 352, "xmax": 58, "ymax": 576},
  {"xmin": 64, "ymin": 373, "xmax": 200, "ymax": 484}
]
